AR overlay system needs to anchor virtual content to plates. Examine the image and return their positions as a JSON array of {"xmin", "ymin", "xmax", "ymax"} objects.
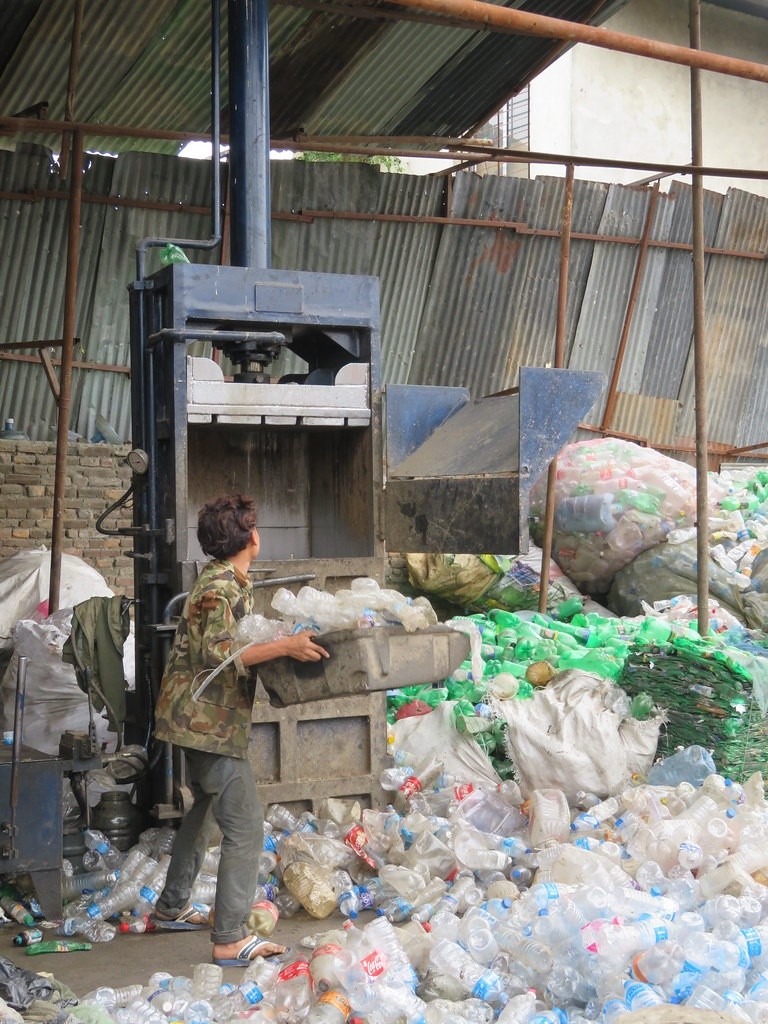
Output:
[{"xmin": 50, "ymin": 424, "xmax": 83, "ymax": 440}]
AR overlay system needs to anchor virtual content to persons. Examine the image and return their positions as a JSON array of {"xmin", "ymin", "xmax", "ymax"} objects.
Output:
[{"xmin": 152, "ymin": 493, "xmax": 331, "ymax": 966}]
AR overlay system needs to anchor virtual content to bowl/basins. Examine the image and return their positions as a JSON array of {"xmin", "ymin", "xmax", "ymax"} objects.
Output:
[
  {"xmin": 0, "ymin": 431, "xmax": 30, "ymax": 440},
  {"xmin": 96, "ymin": 412, "xmax": 122, "ymax": 444}
]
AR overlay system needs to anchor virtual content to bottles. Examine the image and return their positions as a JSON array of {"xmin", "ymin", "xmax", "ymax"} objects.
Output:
[
  {"xmin": 5, "ymin": 418, "xmax": 13, "ymax": 430},
  {"xmin": 159, "ymin": 242, "xmax": 192, "ymax": 266},
  {"xmin": 0, "ymin": 438, "xmax": 767, "ymax": 1023}
]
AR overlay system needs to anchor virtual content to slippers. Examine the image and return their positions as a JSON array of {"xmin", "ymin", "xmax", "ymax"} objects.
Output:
[
  {"xmin": 213, "ymin": 936, "xmax": 292, "ymax": 967},
  {"xmin": 149, "ymin": 905, "xmax": 210, "ymax": 930}
]
